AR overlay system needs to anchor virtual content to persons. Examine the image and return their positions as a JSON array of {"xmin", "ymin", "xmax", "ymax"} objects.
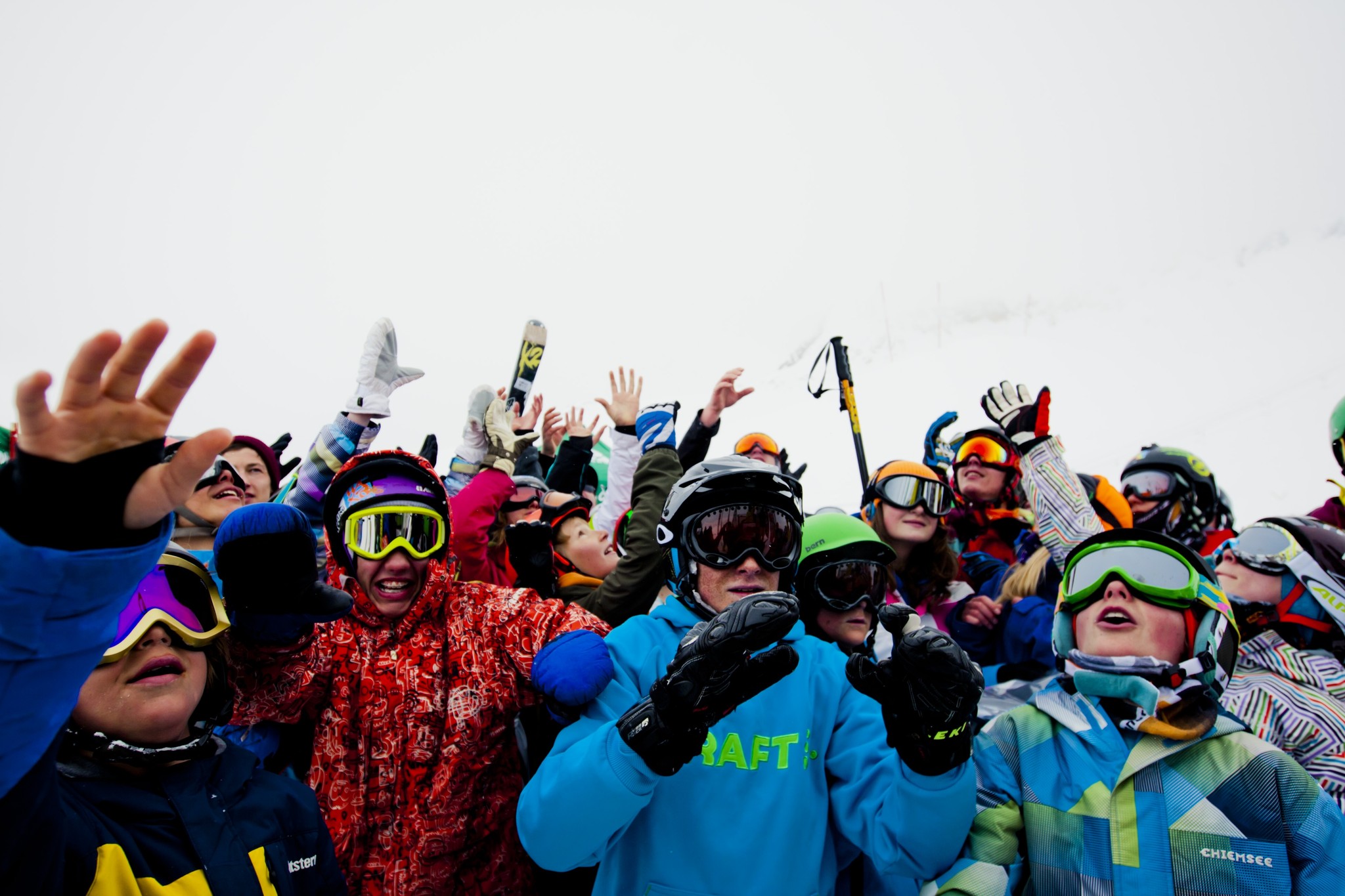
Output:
[
  {"xmin": 0, "ymin": 320, "xmax": 347, "ymax": 896},
  {"xmin": 211, "ymin": 451, "xmax": 614, "ymax": 896},
  {"xmin": 3, "ymin": 315, "xmax": 1344, "ymax": 813},
  {"xmin": 517, "ymin": 451, "xmax": 985, "ymax": 896},
  {"xmin": 921, "ymin": 529, "xmax": 1345, "ymax": 896}
]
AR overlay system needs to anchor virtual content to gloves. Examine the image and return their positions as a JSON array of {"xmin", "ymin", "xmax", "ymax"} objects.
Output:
[
  {"xmin": 531, "ymin": 630, "xmax": 613, "ymax": 732},
  {"xmin": 213, "ymin": 502, "xmax": 354, "ymax": 649},
  {"xmin": 340, "ymin": 317, "xmax": 425, "ymax": 419},
  {"xmin": 505, "ymin": 519, "xmax": 559, "ymax": 601},
  {"xmin": 845, "ymin": 602, "xmax": 984, "ymax": 776},
  {"xmin": 615, "ymin": 591, "xmax": 799, "ymax": 776}
]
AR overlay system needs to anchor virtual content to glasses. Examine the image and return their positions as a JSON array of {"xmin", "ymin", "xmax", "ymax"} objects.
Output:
[
  {"xmin": 342, "ymin": 506, "xmax": 445, "ymax": 560},
  {"xmin": 657, "ymin": 502, "xmax": 803, "ymax": 574},
  {"xmin": 806, "ymin": 559, "xmax": 889, "ymax": 613},
  {"xmin": 1210, "ymin": 521, "xmax": 1306, "ymax": 576},
  {"xmin": 94, "ymin": 553, "xmax": 231, "ymax": 666},
  {"xmin": 1053, "ymin": 540, "xmax": 1241, "ymax": 647}
]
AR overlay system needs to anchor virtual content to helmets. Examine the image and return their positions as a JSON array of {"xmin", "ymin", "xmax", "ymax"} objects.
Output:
[
  {"xmin": 153, "ymin": 380, "xmax": 1344, "ymax": 521},
  {"xmin": 789, "ymin": 512, "xmax": 898, "ymax": 641},
  {"xmin": 1255, "ymin": 516, "xmax": 1345, "ymax": 668},
  {"xmin": 1050, "ymin": 528, "xmax": 1240, "ymax": 700}
]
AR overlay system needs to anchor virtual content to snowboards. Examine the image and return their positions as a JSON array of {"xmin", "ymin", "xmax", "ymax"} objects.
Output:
[{"xmin": 504, "ymin": 317, "xmax": 547, "ymax": 424}]
